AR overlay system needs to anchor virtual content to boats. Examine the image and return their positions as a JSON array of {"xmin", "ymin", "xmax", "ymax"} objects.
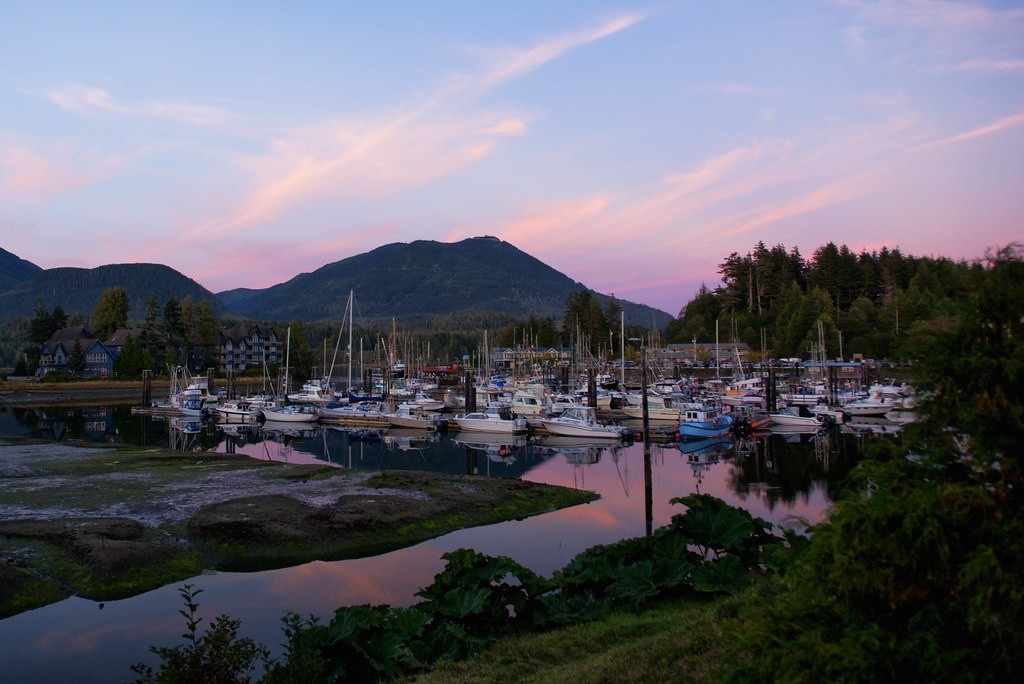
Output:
[
  {"xmin": 846, "ymin": 411, "xmax": 907, "ymax": 435},
  {"xmin": 381, "ymin": 426, "xmax": 441, "ymax": 452},
  {"xmin": 540, "ymin": 406, "xmax": 632, "ymax": 441},
  {"xmin": 386, "ymin": 404, "xmax": 441, "ymax": 429},
  {"xmin": 760, "ymin": 420, "xmax": 830, "ymax": 437},
  {"xmin": 675, "ymin": 436, "xmax": 735, "ymax": 471},
  {"xmin": 449, "ymin": 429, "xmax": 527, "ymax": 467},
  {"xmin": 538, "ymin": 433, "xmax": 634, "ymax": 465},
  {"xmin": 845, "ymin": 397, "xmax": 895, "ymax": 416},
  {"xmin": 152, "ymin": 366, "xmax": 271, "ymax": 421},
  {"xmin": 214, "ymin": 422, "xmax": 259, "ymax": 441},
  {"xmin": 678, "ymin": 409, "xmax": 732, "ymax": 438},
  {"xmin": 766, "ymin": 405, "xmax": 837, "ymax": 425},
  {"xmin": 451, "ymin": 406, "xmax": 528, "ymax": 433},
  {"xmin": 170, "ymin": 416, "xmax": 204, "ymax": 434}
]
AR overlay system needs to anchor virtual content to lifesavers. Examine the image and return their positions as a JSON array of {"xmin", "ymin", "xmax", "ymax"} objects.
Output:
[{"xmin": 713, "ymin": 419, "xmax": 718, "ymax": 429}]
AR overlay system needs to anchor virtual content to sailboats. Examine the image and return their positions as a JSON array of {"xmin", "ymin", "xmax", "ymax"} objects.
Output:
[
  {"xmin": 293, "ymin": 312, "xmax": 928, "ymax": 419},
  {"xmin": 319, "ymin": 289, "xmax": 394, "ymax": 424},
  {"xmin": 257, "ymin": 328, "xmax": 322, "ymax": 423},
  {"xmin": 260, "ymin": 418, "xmax": 319, "ymax": 463},
  {"xmin": 319, "ymin": 416, "xmax": 391, "ymax": 469}
]
